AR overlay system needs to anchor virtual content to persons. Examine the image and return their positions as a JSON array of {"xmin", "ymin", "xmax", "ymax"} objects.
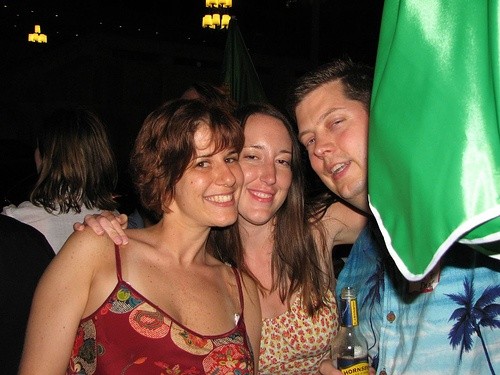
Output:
[
  {"xmin": 0, "ymin": 56, "xmax": 499, "ymax": 375},
  {"xmin": 17, "ymin": 97, "xmax": 263, "ymax": 375}
]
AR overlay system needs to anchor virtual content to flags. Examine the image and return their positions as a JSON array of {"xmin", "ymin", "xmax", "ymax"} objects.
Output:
[
  {"xmin": 222, "ymin": 20, "xmax": 264, "ymax": 106},
  {"xmin": 366, "ymin": 0, "xmax": 500, "ymax": 282}
]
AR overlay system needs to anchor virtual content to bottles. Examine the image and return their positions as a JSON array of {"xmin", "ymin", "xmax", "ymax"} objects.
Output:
[{"xmin": 331, "ymin": 287, "xmax": 370, "ymax": 375}]
